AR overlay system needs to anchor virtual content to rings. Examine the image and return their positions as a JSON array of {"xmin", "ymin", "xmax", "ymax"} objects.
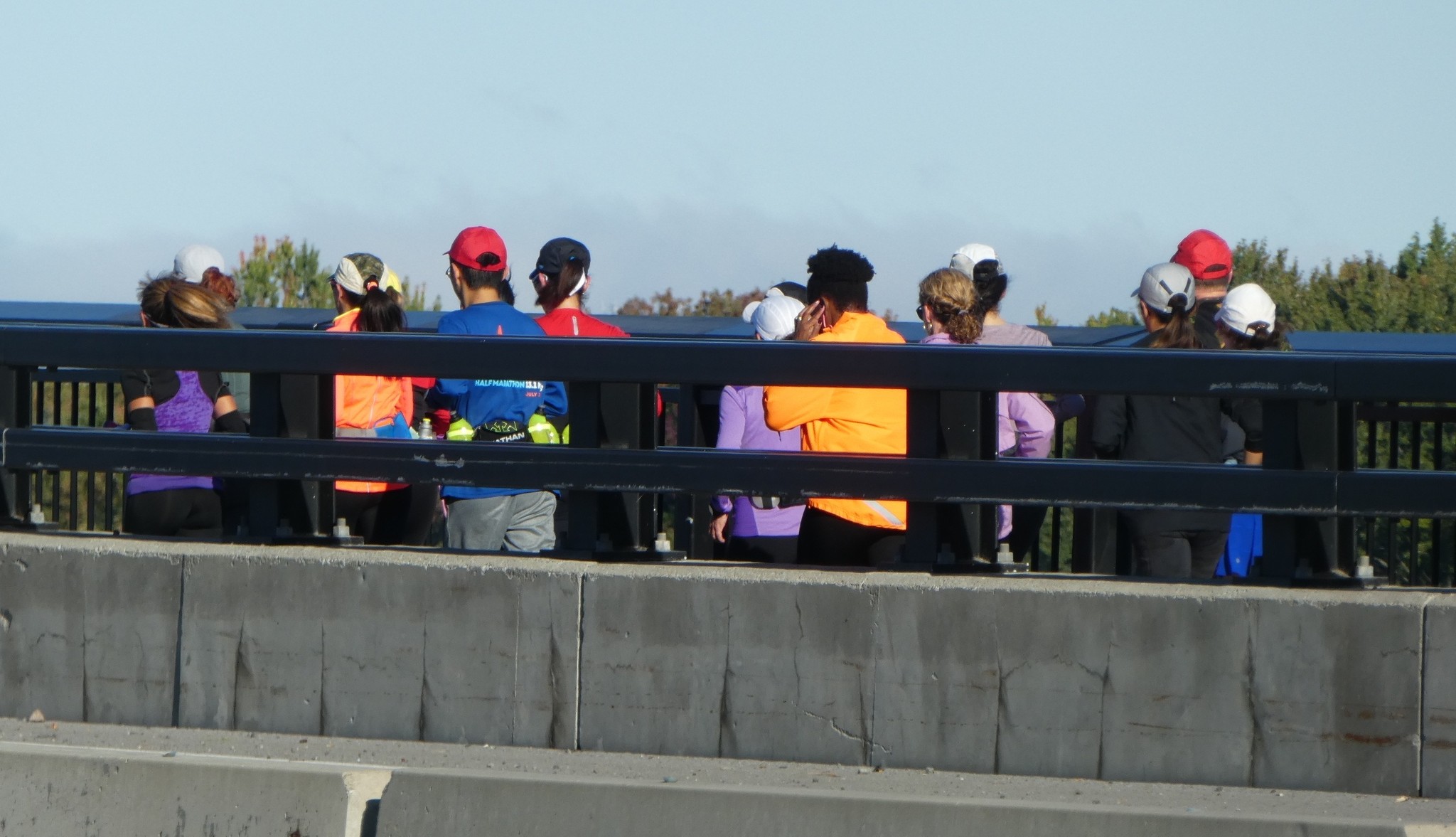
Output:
[{"xmin": 794, "ymin": 317, "xmax": 802, "ymax": 322}]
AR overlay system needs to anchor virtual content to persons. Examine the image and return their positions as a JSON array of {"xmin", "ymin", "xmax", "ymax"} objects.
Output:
[
  {"xmin": 1169, "ymin": 230, "xmax": 1234, "ymax": 352},
  {"xmin": 761, "ymin": 243, "xmax": 907, "ymax": 567},
  {"xmin": 1213, "ymin": 283, "xmax": 1329, "ymax": 588},
  {"xmin": 322, "ymin": 252, "xmax": 440, "ymax": 546},
  {"xmin": 119, "ymin": 272, "xmax": 248, "ymax": 544},
  {"xmin": 425, "ymin": 225, "xmax": 569, "ymax": 554},
  {"xmin": 914, "ymin": 267, "xmax": 1055, "ymax": 539},
  {"xmin": 172, "ymin": 243, "xmax": 250, "ymax": 544},
  {"xmin": 708, "ymin": 295, "xmax": 808, "ymax": 562},
  {"xmin": 1090, "ymin": 261, "xmax": 1265, "ymax": 579},
  {"xmin": 530, "ymin": 238, "xmax": 662, "ymax": 553},
  {"xmin": 763, "ymin": 281, "xmax": 808, "ymax": 305},
  {"xmin": 949, "ymin": 242, "xmax": 1064, "ymax": 562}
]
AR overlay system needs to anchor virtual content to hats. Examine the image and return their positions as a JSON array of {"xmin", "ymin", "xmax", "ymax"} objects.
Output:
[
  {"xmin": 1130, "ymin": 261, "xmax": 1196, "ymax": 315},
  {"xmin": 1169, "ymin": 227, "xmax": 1233, "ymax": 281},
  {"xmin": 1213, "ymin": 281, "xmax": 1276, "ymax": 340},
  {"xmin": 442, "ymin": 225, "xmax": 507, "ymax": 272},
  {"xmin": 947, "ymin": 242, "xmax": 1005, "ymax": 282},
  {"xmin": 764, "ymin": 280, "xmax": 807, "ymax": 307},
  {"xmin": 386, "ymin": 267, "xmax": 403, "ymax": 295},
  {"xmin": 325, "ymin": 252, "xmax": 389, "ymax": 296},
  {"xmin": 527, "ymin": 236, "xmax": 591, "ymax": 280},
  {"xmin": 172, "ymin": 244, "xmax": 224, "ymax": 284},
  {"xmin": 740, "ymin": 294, "xmax": 814, "ymax": 340}
]
